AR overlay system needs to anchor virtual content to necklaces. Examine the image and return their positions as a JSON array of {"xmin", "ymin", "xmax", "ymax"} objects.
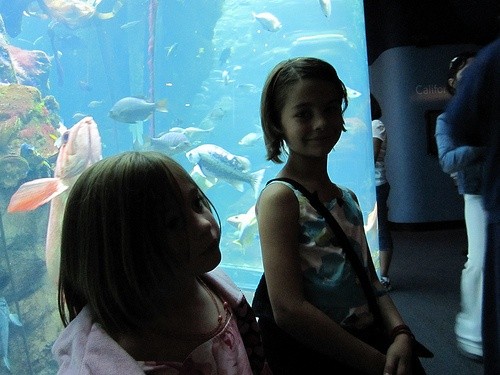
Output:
[{"xmin": 155, "ymin": 273, "xmax": 229, "ymax": 338}]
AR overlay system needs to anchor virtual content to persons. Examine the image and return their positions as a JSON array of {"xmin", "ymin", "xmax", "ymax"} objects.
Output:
[
  {"xmin": 53, "ymin": 152, "xmax": 270, "ymax": 375},
  {"xmin": 369, "ymin": 93, "xmax": 393, "ymax": 293},
  {"xmin": 446, "ymin": 38, "xmax": 500, "ymax": 375},
  {"xmin": 251, "ymin": 57, "xmax": 435, "ymax": 375},
  {"xmin": 435, "ymin": 51, "xmax": 487, "ymax": 360}
]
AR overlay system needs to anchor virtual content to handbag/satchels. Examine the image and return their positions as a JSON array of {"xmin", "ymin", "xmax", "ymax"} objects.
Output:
[{"xmin": 252, "ymin": 180, "xmax": 427, "ymax": 375}]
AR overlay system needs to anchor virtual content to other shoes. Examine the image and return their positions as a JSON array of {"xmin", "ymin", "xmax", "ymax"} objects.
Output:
[{"xmin": 457, "ymin": 345, "xmax": 484, "ymax": 363}]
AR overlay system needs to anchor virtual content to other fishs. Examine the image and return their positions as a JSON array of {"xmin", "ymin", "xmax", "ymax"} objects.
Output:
[{"xmin": 0, "ymin": 0, "xmax": 382, "ymax": 372}]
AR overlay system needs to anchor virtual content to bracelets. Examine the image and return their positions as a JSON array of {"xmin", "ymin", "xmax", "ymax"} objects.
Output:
[{"xmin": 391, "ymin": 324, "xmax": 415, "ymax": 345}]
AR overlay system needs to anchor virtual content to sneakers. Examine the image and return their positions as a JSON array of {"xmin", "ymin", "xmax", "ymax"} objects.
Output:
[{"xmin": 381, "ymin": 276, "xmax": 391, "ymax": 291}]
